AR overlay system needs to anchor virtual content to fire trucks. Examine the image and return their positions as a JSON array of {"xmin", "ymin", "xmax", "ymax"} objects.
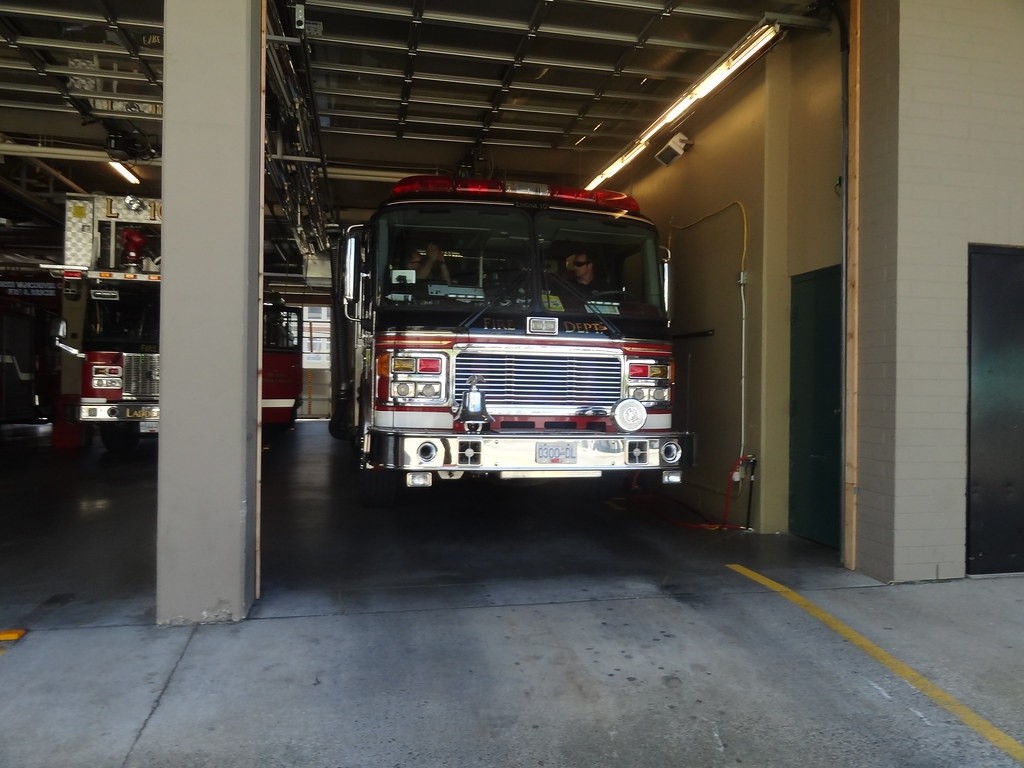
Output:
[
  {"xmin": 40, "ymin": 192, "xmax": 302, "ymax": 461},
  {"xmin": 329, "ymin": 157, "xmax": 695, "ymax": 499}
]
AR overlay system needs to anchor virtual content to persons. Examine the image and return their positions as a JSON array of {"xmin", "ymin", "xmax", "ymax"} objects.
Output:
[
  {"xmin": 561, "ymin": 249, "xmax": 607, "ymax": 296},
  {"xmin": 406, "ymin": 242, "xmax": 452, "ymax": 285}
]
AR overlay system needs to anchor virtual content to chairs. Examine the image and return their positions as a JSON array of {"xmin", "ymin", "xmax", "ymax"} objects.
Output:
[{"xmin": 533, "ymin": 258, "xmax": 603, "ymax": 312}]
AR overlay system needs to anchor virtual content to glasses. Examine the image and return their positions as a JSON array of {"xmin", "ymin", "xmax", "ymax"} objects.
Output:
[{"xmin": 573, "ymin": 261, "xmax": 589, "ymax": 267}]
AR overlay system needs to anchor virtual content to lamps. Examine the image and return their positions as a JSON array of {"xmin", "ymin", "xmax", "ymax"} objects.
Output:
[{"xmin": 654, "ymin": 132, "xmax": 693, "ymax": 167}]
